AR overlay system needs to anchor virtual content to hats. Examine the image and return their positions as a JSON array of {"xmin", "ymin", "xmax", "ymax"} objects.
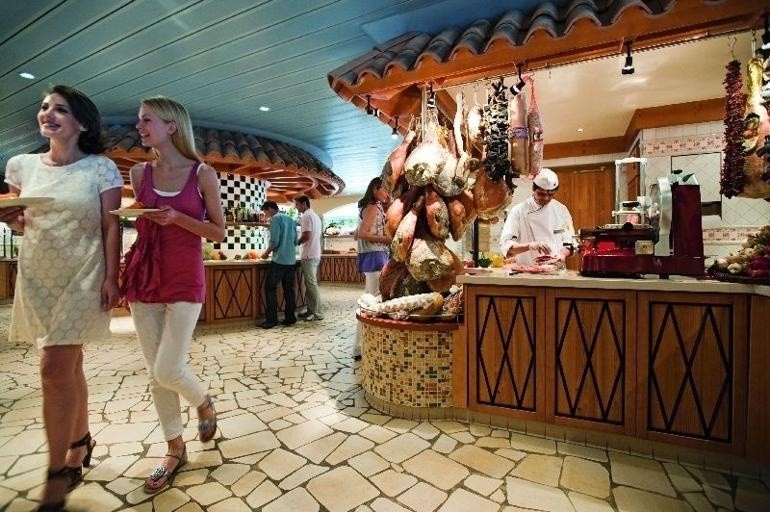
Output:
[{"xmin": 533, "ymin": 168, "xmax": 559, "ymax": 191}]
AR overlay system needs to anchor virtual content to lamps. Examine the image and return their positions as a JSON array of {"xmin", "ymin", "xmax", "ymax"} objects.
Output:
[
  {"xmin": 365, "ymin": 95, "xmax": 379, "ymax": 117},
  {"xmin": 391, "ymin": 116, "xmax": 400, "ymax": 141},
  {"xmin": 427, "ymin": 81, "xmax": 437, "ymax": 108},
  {"xmin": 622, "ymin": 39, "xmax": 635, "ymax": 74},
  {"xmin": 509, "ymin": 63, "xmax": 525, "ymax": 96}
]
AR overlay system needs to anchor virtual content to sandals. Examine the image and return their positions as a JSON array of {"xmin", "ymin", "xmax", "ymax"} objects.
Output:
[
  {"xmin": 198, "ymin": 396, "xmax": 217, "ymax": 443},
  {"xmin": 143, "ymin": 442, "xmax": 188, "ymax": 493}
]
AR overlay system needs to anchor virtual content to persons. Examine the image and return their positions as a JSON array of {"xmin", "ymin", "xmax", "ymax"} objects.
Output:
[
  {"xmin": 500, "ymin": 168, "xmax": 580, "ymax": 269},
  {"xmin": 352, "ymin": 177, "xmax": 392, "ymax": 360},
  {"xmin": 294, "ymin": 196, "xmax": 324, "ymax": 321},
  {"xmin": 0, "ymin": 85, "xmax": 119, "ymax": 512},
  {"xmin": 260, "ymin": 200, "xmax": 297, "ymax": 328},
  {"xmin": 119, "ymin": 96, "xmax": 225, "ymax": 494}
]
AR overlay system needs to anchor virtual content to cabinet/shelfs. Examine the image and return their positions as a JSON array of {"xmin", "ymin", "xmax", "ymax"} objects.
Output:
[
  {"xmin": 117, "ymin": 221, "xmax": 277, "ymax": 266},
  {"xmin": 322, "ymin": 234, "xmax": 359, "ymax": 255}
]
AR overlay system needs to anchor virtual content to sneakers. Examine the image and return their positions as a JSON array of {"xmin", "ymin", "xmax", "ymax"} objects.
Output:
[
  {"xmin": 299, "ymin": 312, "xmax": 312, "ymax": 317},
  {"xmin": 279, "ymin": 316, "xmax": 297, "ymax": 325},
  {"xmin": 256, "ymin": 320, "xmax": 278, "ymax": 329},
  {"xmin": 306, "ymin": 313, "xmax": 324, "ymax": 321}
]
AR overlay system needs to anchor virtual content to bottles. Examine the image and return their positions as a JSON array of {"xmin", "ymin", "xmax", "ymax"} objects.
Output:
[
  {"xmin": 466, "ymin": 254, "xmax": 474, "ymax": 267},
  {"xmin": 478, "ymin": 248, "xmax": 493, "ymax": 268},
  {"xmin": 221, "ymin": 203, "xmax": 271, "ymax": 224},
  {"xmin": 491, "ymin": 238, "xmax": 504, "ymax": 267},
  {"xmin": 279, "ymin": 206, "xmax": 302, "ymax": 222}
]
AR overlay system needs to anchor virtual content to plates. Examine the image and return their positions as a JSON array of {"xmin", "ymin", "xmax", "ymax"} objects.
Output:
[
  {"xmin": 108, "ymin": 208, "xmax": 164, "ymax": 217},
  {"xmin": 0, "ymin": 197, "xmax": 57, "ymax": 208}
]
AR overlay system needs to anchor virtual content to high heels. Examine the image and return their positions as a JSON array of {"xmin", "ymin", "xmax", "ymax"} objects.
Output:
[
  {"xmin": 34, "ymin": 469, "xmax": 67, "ymax": 511},
  {"xmin": 62, "ymin": 431, "xmax": 97, "ymax": 494}
]
